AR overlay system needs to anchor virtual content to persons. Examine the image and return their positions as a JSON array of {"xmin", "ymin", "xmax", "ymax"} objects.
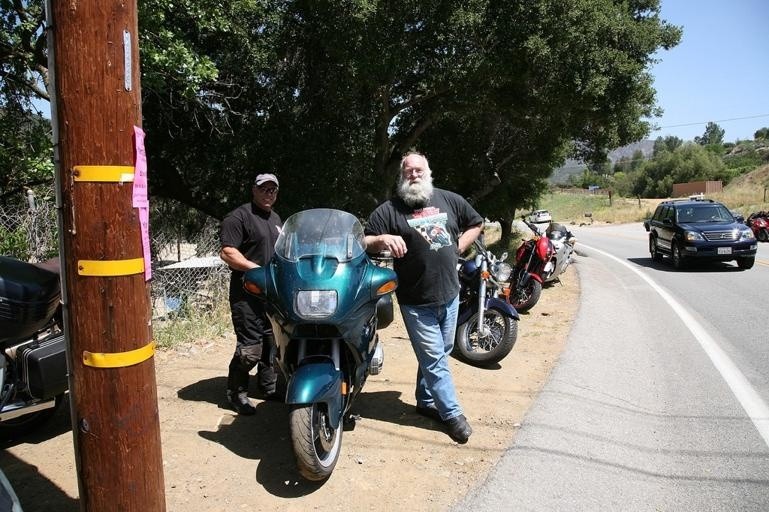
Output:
[
  {"xmin": 359, "ymin": 149, "xmax": 486, "ymax": 444},
  {"xmin": 216, "ymin": 171, "xmax": 286, "ymax": 418}
]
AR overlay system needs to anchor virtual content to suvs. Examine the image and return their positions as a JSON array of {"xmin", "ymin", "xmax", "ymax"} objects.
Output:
[{"xmin": 648, "ymin": 195, "xmax": 757, "ymax": 270}]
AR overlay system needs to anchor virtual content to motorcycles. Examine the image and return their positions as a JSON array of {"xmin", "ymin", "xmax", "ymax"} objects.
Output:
[
  {"xmin": 748, "ymin": 210, "xmax": 769, "ymax": 242},
  {"xmin": 454, "ymin": 215, "xmax": 575, "ymax": 367},
  {"xmin": 0, "ymin": 257, "xmax": 71, "ymax": 421},
  {"xmin": 239, "ymin": 208, "xmax": 400, "ymax": 483}
]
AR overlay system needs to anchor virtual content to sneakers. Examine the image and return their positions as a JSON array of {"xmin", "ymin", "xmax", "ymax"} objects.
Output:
[
  {"xmin": 446, "ymin": 414, "xmax": 471, "ymax": 441},
  {"xmin": 416, "ymin": 404, "xmax": 442, "ymax": 420},
  {"xmin": 261, "ymin": 390, "xmax": 283, "ymax": 401},
  {"xmin": 228, "ymin": 396, "xmax": 256, "ymax": 415}
]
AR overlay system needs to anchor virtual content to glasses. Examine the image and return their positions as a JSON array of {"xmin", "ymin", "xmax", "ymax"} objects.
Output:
[{"xmin": 261, "ymin": 186, "xmax": 278, "ymax": 193}]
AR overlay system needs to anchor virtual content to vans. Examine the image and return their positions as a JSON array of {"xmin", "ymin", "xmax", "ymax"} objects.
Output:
[{"xmin": 529, "ymin": 210, "xmax": 551, "ymax": 223}]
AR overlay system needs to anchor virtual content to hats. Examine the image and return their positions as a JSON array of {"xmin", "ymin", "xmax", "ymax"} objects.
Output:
[{"xmin": 254, "ymin": 173, "xmax": 279, "ymax": 187}]
